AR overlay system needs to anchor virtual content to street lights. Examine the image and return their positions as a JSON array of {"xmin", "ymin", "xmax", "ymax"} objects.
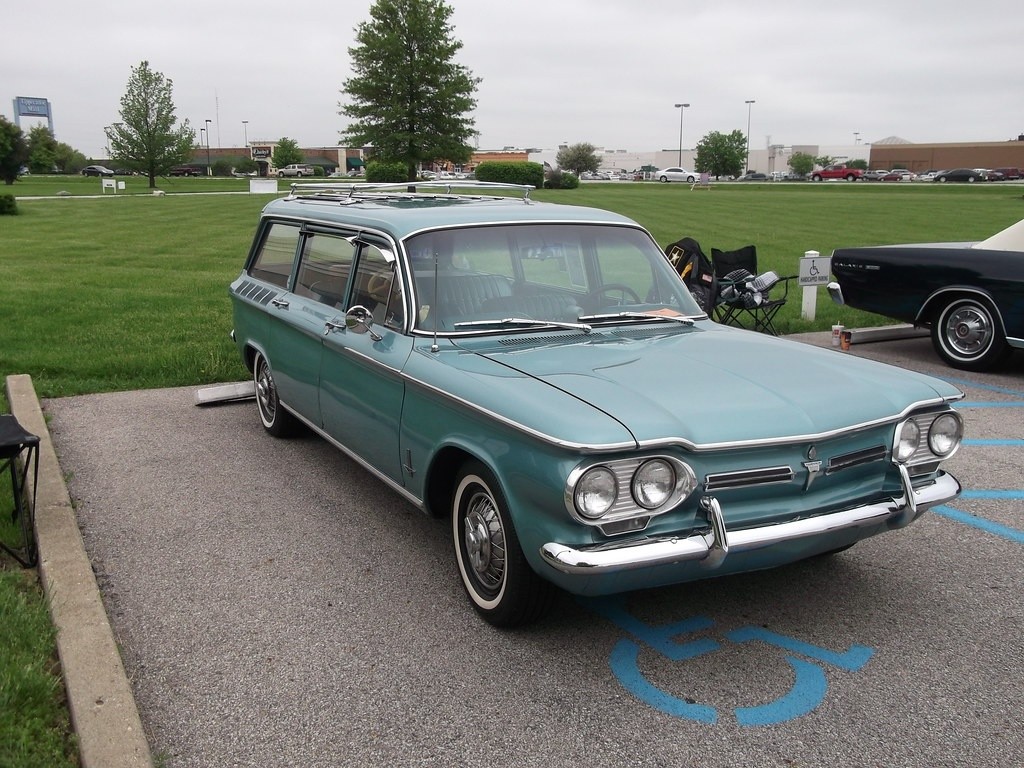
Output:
[
  {"xmin": 744, "ymin": 100, "xmax": 755, "ymax": 174},
  {"xmin": 241, "ymin": 121, "xmax": 249, "ymax": 147},
  {"xmin": 674, "ymin": 103, "xmax": 690, "ymax": 167},
  {"xmin": 205, "ymin": 120, "xmax": 212, "ymax": 176},
  {"xmin": 201, "ymin": 129, "xmax": 205, "ymax": 147}
]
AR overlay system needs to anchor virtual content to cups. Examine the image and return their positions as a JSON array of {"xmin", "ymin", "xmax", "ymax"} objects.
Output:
[
  {"xmin": 831, "ymin": 325, "xmax": 844, "ymax": 346},
  {"xmin": 840, "ymin": 331, "xmax": 851, "ymax": 350}
]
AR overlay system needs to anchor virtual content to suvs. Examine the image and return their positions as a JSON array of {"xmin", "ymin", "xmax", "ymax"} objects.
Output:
[{"xmin": 276, "ymin": 164, "xmax": 315, "ymax": 178}]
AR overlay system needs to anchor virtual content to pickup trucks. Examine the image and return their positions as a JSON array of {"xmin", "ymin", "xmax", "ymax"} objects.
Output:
[
  {"xmin": 167, "ymin": 164, "xmax": 202, "ymax": 177},
  {"xmin": 811, "ymin": 163, "xmax": 864, "ymax": 182}
]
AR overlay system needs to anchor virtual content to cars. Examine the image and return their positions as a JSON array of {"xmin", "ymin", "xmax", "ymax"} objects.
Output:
[
  {"xmin": 327, "ymin": 169, "xmax": 366, "ymax": 179},
  {"xmin": 82, "ymin": 165, "xmax": 115, "ymax": 178},
  {"xmin": 826, "ymin": 218, "xmax": 1024, "ymax": 372},
  {"xmin": 114, "ymin": 167, "xmax": 150, "ymax": 178},
  {"xmin": 708, "ymin": 161, "xmax": 945, "ymax": 183},
  {"xmin": 975, "ymin": 166, "xmax": 1024, "ymax": 182},
  {"xmin": 227, "ymin": 180, "xmax": 968, "ymax": 631},
  {"xmin": 416, "ymin": 169, "xmax": 475, "ymax": 180},
  {"xmin": 987, "ymin": 172, "xmax": 1005, "ymax": 182},
  {"xmin": 560, "ymin": 169, "xmax": 655, "ymax": 182},
  {"xmin": 878, "ymin": 172, "xmax": 903, "ymax": 182},
  {"xmin": 933, "ymin": 168, "xmax": 981, "ymax": 184},
  {"xmin": 654, "ymin": 167, "xmax": 700, "ymax": 183}
]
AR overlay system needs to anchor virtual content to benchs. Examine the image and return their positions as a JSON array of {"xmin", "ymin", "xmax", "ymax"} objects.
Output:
[{"xmin": 398, "ymin": 274, "xmax": 513, "ymax": 318}]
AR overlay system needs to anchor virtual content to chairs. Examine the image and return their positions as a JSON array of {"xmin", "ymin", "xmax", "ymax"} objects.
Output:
[
  {"xmin": 483, "ymin": 292, "xmax": 575, "ymax": 322},
  {"xmin": 710, "ymin": 246, "xmax": 800, "ymax": 336}
]
